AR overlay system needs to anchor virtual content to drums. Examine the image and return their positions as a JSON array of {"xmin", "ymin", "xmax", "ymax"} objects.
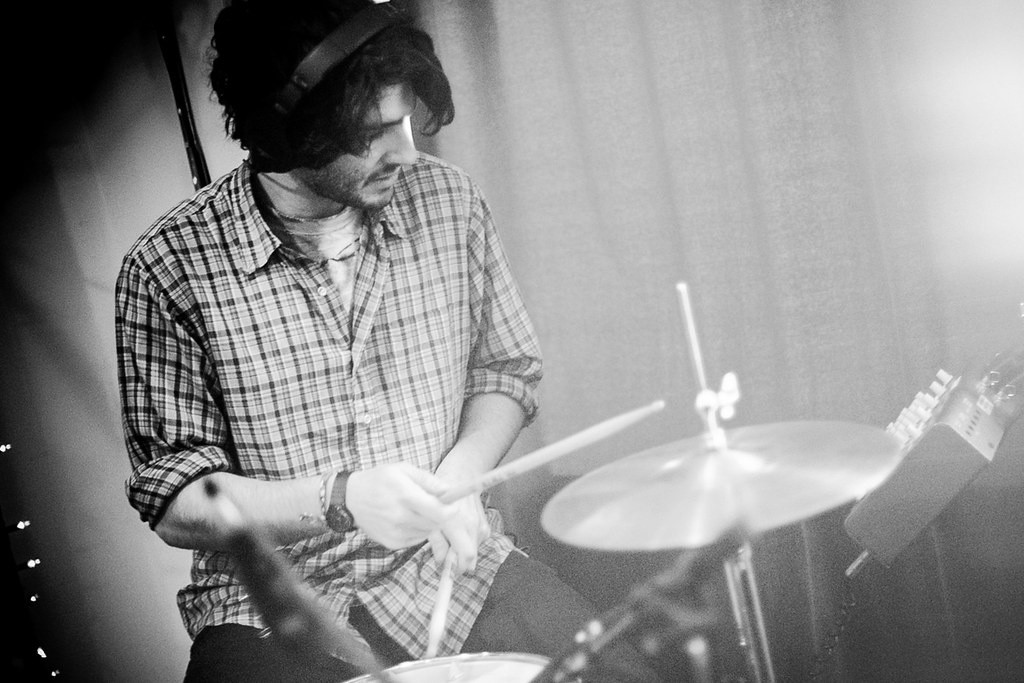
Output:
[{"xmin": 343, "ymin": 648, "xmax": 584, "ymax": 683}]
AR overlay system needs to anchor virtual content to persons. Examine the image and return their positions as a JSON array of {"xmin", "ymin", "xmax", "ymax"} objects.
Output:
[{"xmin": 114, "ymin": 0, "xmax": 660, "ymax": 682}]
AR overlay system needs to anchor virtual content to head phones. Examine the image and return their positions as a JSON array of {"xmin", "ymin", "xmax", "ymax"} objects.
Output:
[{"xmin": 236, "ymin": 1, "xmax": 416, "ymax": 172}]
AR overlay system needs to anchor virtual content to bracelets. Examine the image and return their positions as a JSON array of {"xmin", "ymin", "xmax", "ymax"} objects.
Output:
[{"xmin": 317, "ymin": 470, "xmax": 329, "ymax": 529}]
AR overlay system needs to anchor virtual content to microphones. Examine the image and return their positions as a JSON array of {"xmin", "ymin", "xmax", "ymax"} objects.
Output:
[{"xmin": 205, "ymin": 483, "xmax": 316, "ymax": 653}]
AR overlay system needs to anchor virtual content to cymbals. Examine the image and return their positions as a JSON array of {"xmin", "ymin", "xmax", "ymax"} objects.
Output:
[{"xmin": 539, "ymin": 417, "xmax": 903, "ymax": 554}]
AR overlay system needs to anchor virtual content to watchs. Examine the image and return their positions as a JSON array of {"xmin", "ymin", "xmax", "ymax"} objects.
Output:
[{"xmin": 326, "ymin": 470, "xmax": 358, "ymax": 533}]
